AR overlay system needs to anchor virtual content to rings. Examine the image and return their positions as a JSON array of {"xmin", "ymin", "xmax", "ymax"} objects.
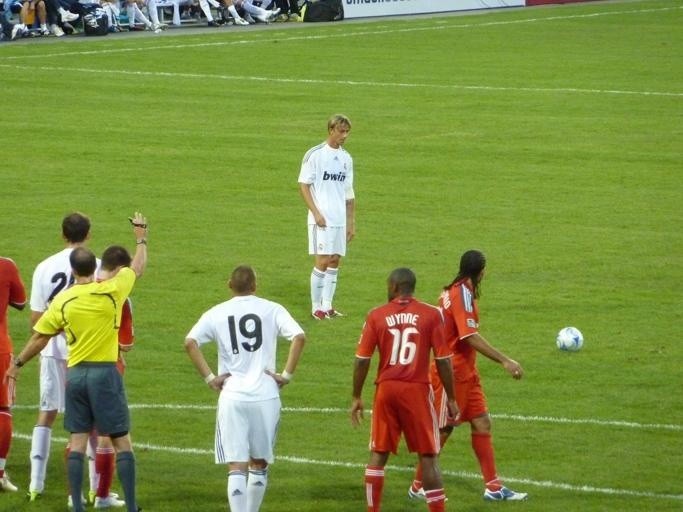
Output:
[{"xmin": 515, "ymin": 370, "xmax": 519, "ymax": 374}]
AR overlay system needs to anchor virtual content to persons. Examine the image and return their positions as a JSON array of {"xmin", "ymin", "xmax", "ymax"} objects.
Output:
[
  {"xmin": 407, "ymin": 249, "xmax": 528, "ymax": 505},
  {"xmin": 2, "ymin": 211, "xmax": 149, "ymax": 512},
  {"xmin": 296, "ymin": 114, "xmax": 356, "ymax": 321},
  {"xmin": 183, "ymin": 263, "xmax": 306, "ymax": 511},
  {"xmin": 350, "ymin": 266, "xmax": 462, "ymax": 511},
  {"xmin": 0, "ymin": 0, "xmax": 299, "ymax": 42},
  {"xmin": 53, "ymin": 246, "xmax": 135, "ymax": 509},
  {"xmin": 27, "ymin": 211, "xmax": 102, "ymax": 504},
  {"xmin": 0, "ymin": 256, "xmax": 28, "ymax": 493}
]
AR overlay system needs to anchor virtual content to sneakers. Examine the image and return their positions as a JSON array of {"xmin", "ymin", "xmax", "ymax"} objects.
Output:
[
  {"xmin": 484, "ymin": 487, "xmax": 529, "ymax": 501},
  {"xmin": 28, "ymin": 492, "xmax": 40, "ymax": 501},
  {"xmin": 68, "ymin": 489, "xmax": 125, "ymax": 507},
  {"xmin": 209, "ymin": 8, "xmax": 299, "ymax": 27},
  {"xmin": 61, "ymin": 12, "xmax": 79, "ymax": 21},
  {"xmin": 1, "ymin": 478, "xmax": 18, "ymax": 491},
  {"xmin": 312, "ymin": 310, "xmax": 342, "ymax": 319},
  {"xmin": 22, "ymin": 25, "xmax": 63, "ymax": 36},
  {"xmin": 129, "ymin": 23, "xmax": 168, "ymax": 30},
  {"xmin": 408, "ymin": 486, "xmax": 447, "ymax": 503}
]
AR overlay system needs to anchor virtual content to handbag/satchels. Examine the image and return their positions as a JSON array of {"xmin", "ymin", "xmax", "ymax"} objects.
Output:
[{"xmin": 83, "ymin": 10, "xmax": 108, "ymax": 35}]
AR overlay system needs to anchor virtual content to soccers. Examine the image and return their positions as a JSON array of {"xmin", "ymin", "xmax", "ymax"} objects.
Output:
[{"xmin": 557, "ymin": 328, "xmax": 584, "ymax": 353}]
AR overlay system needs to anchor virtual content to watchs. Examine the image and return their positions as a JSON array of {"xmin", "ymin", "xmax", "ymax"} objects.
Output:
[{"xmin": 14, "ymin": 356, "xmax": 24, "ymax": 370}]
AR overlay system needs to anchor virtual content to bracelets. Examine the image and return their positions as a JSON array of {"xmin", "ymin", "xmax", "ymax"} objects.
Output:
[
  {"xmin": 136, "ymin": 238, "xmax": 148, "ymax": 246},
  {"xmin": 204, "ymin": 373, "xmax": 216, "ymax": 384},
  {"xmin": 281, "ymin": 370, "xmax": 292, "ymax": 382}
]
